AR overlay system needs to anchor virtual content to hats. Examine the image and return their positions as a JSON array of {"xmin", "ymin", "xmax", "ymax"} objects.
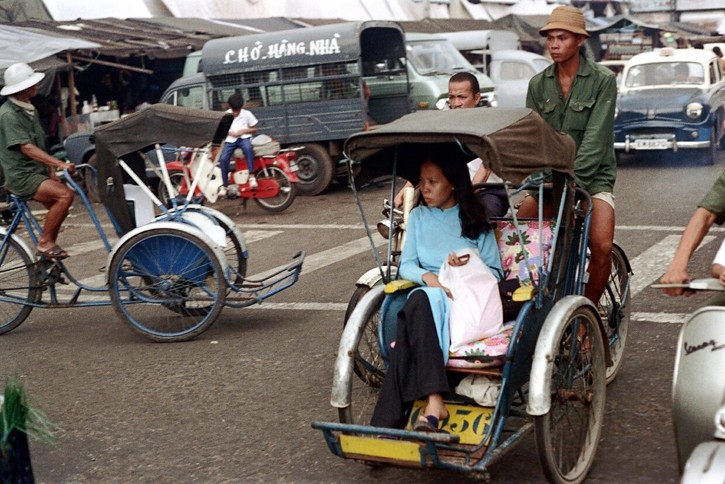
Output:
[
  {"xmin": 0, "ymin": 63, "xmax": 45, "ymax": 95},
  {"xmin": 538, "ymin": 6, "xmax": 590, "ymax": 38}
]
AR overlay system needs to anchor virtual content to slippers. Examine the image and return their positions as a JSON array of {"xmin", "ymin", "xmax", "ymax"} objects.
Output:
[
  {"xmin": 576, "ymin": 335, "xmax": 589, "ymax": 354},
  {"xmin": 413, "ymin": 415, "xmax": 450, "ymax": 434},
  {"xmin": 36, "ymin": 244, "xmax": 70, "ymax": 259}
]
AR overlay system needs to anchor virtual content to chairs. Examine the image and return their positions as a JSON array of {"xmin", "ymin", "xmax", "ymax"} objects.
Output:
[
  {"xmin": 122, "ymin": 186, "xmax": 305, "ymax": 307},
  {"xmin": 311, "ymin": 220, "xmax": 563, "ymax": 476}
]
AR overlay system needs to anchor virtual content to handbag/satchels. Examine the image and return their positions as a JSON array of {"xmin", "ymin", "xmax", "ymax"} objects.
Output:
[{"xmin": 437, "ymin": 248, "xmax": 514, "ymax": 354}]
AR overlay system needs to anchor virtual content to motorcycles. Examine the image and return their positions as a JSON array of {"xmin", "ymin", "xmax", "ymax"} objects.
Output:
[{"xmin": 157, "ymin": 140, "xmax": 305, "ymax": 214}]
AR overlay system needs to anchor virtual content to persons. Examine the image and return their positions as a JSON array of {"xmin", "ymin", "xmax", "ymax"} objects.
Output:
[
  {"xmin": 318, "ymin": 64, "xmax": 370, "ymax": 103},
  {"xmin": 369, "ymin": 145, "xmax": 505, "ymax": 432},
  {"xmin": 670, "ymin": 63, "xmax": 693, "ymax": 84},
  {"xmin": 660, "ymin": 170, "xmax": 725, "ymax": 297},
  {"xmin": 0, "ymin": 63, "xmax": 75, "ymax": 258},
  {"xmin": 394, "ymin": 72, "xmax": 509, "ymax": 227},
  {"xmin": 516, "ymin": 6, "xmax": 618, "ymax": 353},
  {"xmin": 217, "ymin": 94, "xmax": 258, "ymax": 197}
]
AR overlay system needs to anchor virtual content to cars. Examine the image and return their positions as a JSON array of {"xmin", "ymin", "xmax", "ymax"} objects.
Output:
[{"xmin": 612, "ymin": 47, "xmax": 725, "ymax": 165}]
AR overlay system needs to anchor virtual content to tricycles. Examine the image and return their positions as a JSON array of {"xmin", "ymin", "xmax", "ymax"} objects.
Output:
[
  {"xmin": 0, "ymin": 104, "xmax": 307, "ymax": 342},
  {"xmin": 311, "ymin": 107, "xmax": 634, "ymax": 484}
]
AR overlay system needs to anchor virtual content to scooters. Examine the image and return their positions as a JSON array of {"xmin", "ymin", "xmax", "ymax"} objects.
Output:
[{"xmin": 651, "ymin": 277, "xmax": 724, "ymax": 484}]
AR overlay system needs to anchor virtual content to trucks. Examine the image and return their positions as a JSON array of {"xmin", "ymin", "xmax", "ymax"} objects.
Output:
[{"xmin": 61, "ymin": 18, "xmax": 725, "ymax": 196}]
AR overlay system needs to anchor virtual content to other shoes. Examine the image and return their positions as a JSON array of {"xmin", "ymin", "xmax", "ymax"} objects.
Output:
[
  {"xmin": 217, "ymin": 186, "xmax": 228, "ymax": 197},
  {"xmin": 249, "ymin": 176, "xmax": 258, "ymax": 187}
]
